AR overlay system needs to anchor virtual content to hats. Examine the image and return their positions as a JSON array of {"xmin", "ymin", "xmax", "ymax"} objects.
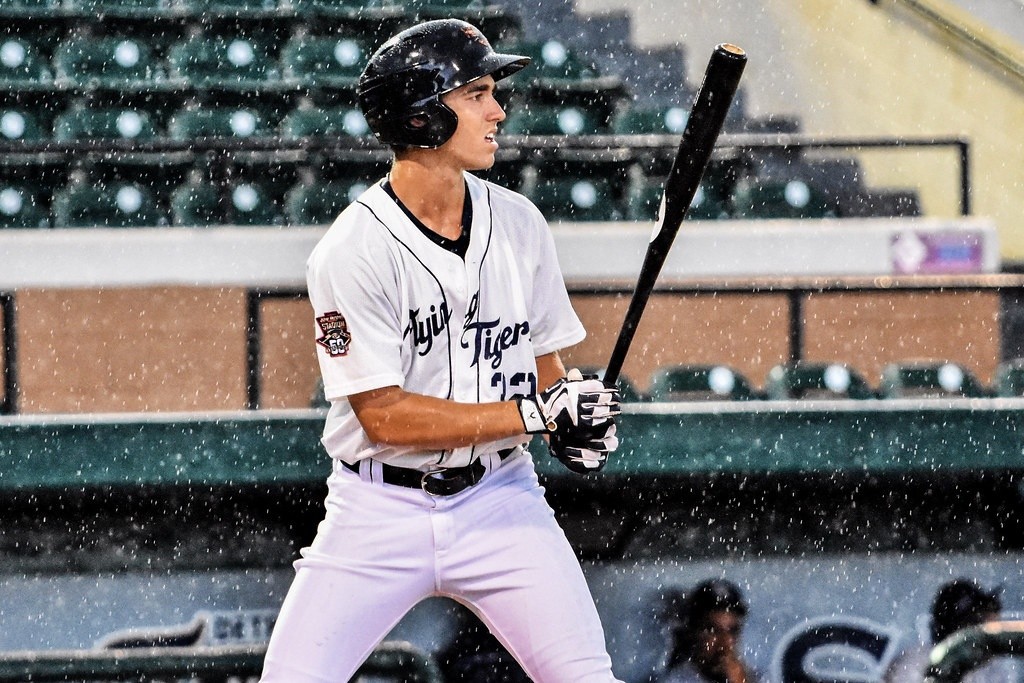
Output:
[
  {"xmin": 693, "ymin": 581, "xmax": 746, "ymax": 611},
  {"xmin": 930, "ymin": 575, "xmax": 1004, "ymax": 643}
]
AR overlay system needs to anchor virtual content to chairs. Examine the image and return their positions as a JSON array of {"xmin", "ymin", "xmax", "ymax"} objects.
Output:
[
  {"xmin": 169, "ymin": 107, "xmax": 261, "ymax": 140},
  {"xmin": 0, "ymin": 34, "xmax": 53, "ymax": 230},
  {"xmin": 878, "ymin": 360, "xmax": 985, "ymax": 399},
  {"xmin": 165, "ymin": 40, "xmax": 268, "ymax": 81},
  {"xmin": 51, "ymin": 36, "xmax": 149, "ymax": 80},
  {"xmin": 628, "ymin": 179, "xmax": 721, "ymax": 219},
  {"xmin": 615, "ymin": 106, "xmax": 686, "ymax": 134},
  {"xmin": 53, "ymin": 109, "xmax": 155, "ymax": 141},
  {"xmin": 736, "ymin": 180, "xmax": 826, "ymax": 219},
  {"xmin": 503, "ymin": 42, "xmax": 582, "ymax": 77},
  {"xmin": 50, "ymin": 185, "xmax": 169, "ymax": 227},
  {"xmin": 505, "ymin": 104, "xmax": 599, "ymax": 135},
  {"xmin": 276, "ymin": 32, "xmax": 367, "ymax": 76},
  {"xmin": 987, "ymin": 359, "xmax": 1024, "ymax": 397},
  {"xmin": 283, "ymin": 182, "xmax": 369, "ymax": 226},
  {"xmin": 533, "ymin": 176, "xmax": 614, "ymax": 220},
  {"xmin": 763, "ymin": 360, "xmax": 874, "ymax": 400},
  {"xmin": 277, "ymin": 105, "xmax": 372, "ymax": 138},
  {"xmin": 647, "ymin": 366, "xmax": 760, "ymax": 400},
  {"xmin": 172, "ymin": 181, "xmax": 280, "ymax": 226}
]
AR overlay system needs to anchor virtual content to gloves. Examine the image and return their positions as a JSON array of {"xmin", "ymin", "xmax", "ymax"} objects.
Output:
[
  {"xmin": 547, "ymin": 429, "xmax": 618, "ymax": 473},
  {"xmin": 517, "ymin": 368, "xmax": 620, "ymax": 434}
]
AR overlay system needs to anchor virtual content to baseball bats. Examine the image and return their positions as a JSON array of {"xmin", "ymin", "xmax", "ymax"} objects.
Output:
[{"xmin": 603, "ymin": 41, "xmax": 749, "ymax": 386}]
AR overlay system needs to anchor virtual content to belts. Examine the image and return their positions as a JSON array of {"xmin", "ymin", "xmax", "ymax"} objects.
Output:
[{"xmin": 340, "ymin": 447, "xmax": 516, "ymax": 497}]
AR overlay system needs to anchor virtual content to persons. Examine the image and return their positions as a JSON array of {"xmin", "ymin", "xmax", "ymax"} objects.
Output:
[
  {"xmin": 255, "ymin": 18, "xmax": 626, "ymax": 683},
  {"xmin": 643, "ymin": 578, "xmax": 1024, "ymax": 683}
]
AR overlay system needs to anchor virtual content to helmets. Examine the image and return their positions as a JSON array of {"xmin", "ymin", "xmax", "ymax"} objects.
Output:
[{"xmin": 356, "ymin": 19, "xmax": 531, "ymax": 151}]
